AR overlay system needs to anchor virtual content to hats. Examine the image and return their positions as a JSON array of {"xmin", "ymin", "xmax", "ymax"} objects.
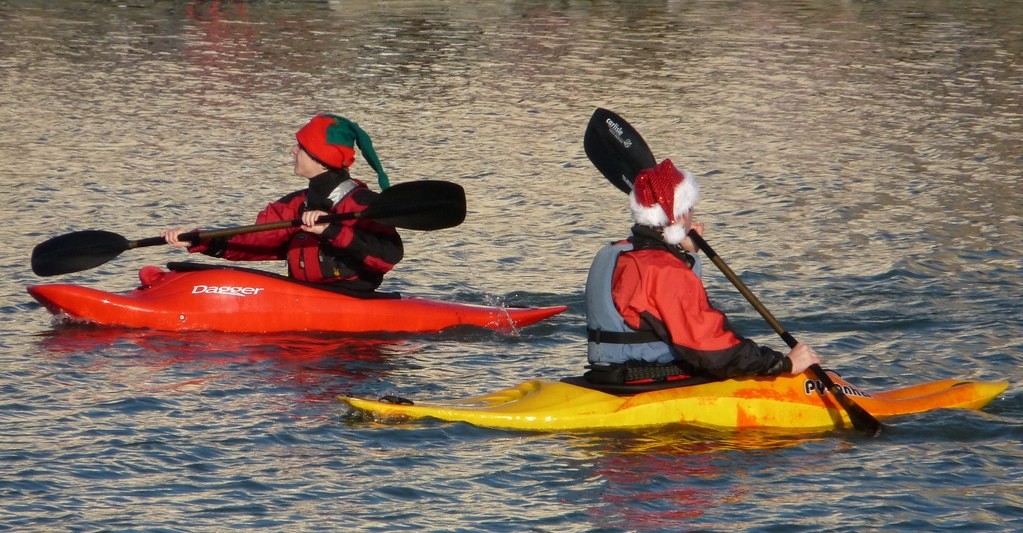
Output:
[
  {"xmin": 628, "ymin": 158, "xmax": 699, "ymax": 244},
  {"xmin": 295, "ymin": 114, "xmax": 390, "ymax": 192}
]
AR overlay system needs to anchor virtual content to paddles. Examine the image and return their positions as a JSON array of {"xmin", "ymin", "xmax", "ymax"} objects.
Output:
[
  {"xmin": 28, "ymin": 178, "xmax": 469, "ymax": 278},
  {"xmin": 580, "ymin": 103, "xmax": 887, "ymax": 435}
]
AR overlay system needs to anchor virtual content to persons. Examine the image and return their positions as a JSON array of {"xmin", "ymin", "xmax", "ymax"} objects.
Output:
[
  {"xmin": 160, "ymin": 113, "xmax": 404, "ymax": 291},
  {"xmin": 584, "ymin": 159, "xmax": 819, "ymax": 384}
]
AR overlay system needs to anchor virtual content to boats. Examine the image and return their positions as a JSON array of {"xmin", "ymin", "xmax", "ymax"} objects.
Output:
[
  {"xmin": 24, "ymin": 250, "xmax": 568, "ymax": 339},
  {"xmin": 335, "ymin": 349, "xmax": 1009, "ymax": 433}
]
